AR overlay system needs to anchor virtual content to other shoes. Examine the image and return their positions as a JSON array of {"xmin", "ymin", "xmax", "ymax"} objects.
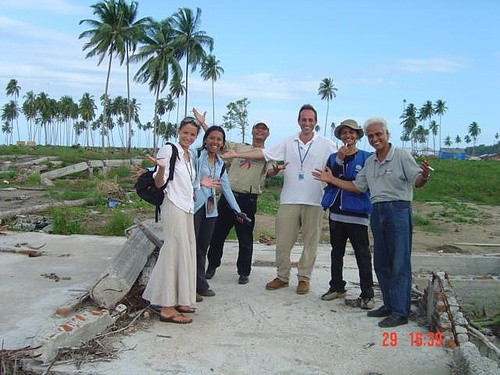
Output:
[
  {"xmin": 198, "ymin": 289, "xmax": 215, "ymax": 296},
  {"xmin": 321, "ymin": 290, "xmax": 345, "ymax": 300},
  {"xmin": 297, "ymin": 281, "xmax": 309, "ymax": 294},
  {"xmin": 360, "ymin": 297, "xmax": 375, "ymax": 309},
  {"xmin": 367, "ymin": 306, "xmax": 388, "ymax": 317},
  {"xmin": 196, "ymin": 293, "xmax": 203, "ymax": 302},
  {"xmin": 379, "ymin": 317, "xmax": 409, "ymax": 327},
  {"xmin": 206, "ymin": 264, "xmax": 220, "ymax": 279},
  {"xmin": 238, "ymin": 275, "xmax": 249, "ymax": 284},
  {"xmin": 266, "ymin": 278, "xmax": 290, "ymax": 290}
]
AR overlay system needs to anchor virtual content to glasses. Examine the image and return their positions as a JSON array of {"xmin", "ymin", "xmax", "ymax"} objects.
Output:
[{"xmin": 181, "ymin": 117, "xmax": 201, "ymax": 126}]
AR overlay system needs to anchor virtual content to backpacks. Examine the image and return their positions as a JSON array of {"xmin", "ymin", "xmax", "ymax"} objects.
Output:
[{"xmin": 135, "ymin": 141, "xmax": 180, "ymax": 206}]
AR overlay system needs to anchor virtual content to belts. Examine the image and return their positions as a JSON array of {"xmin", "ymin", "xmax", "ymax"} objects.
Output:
[{"xmin": 234, "ymin": 192, "xmax": 257, "ymax": 199}]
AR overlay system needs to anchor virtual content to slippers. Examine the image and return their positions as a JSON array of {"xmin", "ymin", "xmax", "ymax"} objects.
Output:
[
  {"xmin": 160, "ymin": 312, "xmax": 192, "ymax": 324},
  {"xmin": 176, "ymin": 305, "xmax": 195, "ymax": 313}
]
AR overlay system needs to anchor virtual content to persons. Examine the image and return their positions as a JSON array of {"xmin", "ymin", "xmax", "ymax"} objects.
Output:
[
  {"xmin": 321, "ymin": 118, "xmax": 375, "ymax": 310},
  {"xmin": 147, "ymin": 116, "xmax": 222, "ymax": 324},
  {"xmin": 219, "ymin": 104, "xmax": 338, "ymax": 294},
  {"xmin": 132, "ymin": 125, "xmax": 246, "ymax": 302},
  {"xmin": 310, "ymin": 117, "xmax": 429, "ymax": 327},
  {"xmin": 193, "ymin": 107, "xmax": 289, "ymax": 284}
]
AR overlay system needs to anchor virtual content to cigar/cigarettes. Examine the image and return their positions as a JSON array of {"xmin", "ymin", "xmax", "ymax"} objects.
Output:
[
  {"xmin": 428, "ymin": 166, "xmax": 434, "ymax": 171},
  {"xmin": 346, "ymin": 143, "xmax": 347, "ymax": 147}
]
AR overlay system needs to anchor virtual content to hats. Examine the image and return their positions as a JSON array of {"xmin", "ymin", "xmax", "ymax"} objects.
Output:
[
  {"xmin": 252, "ymin": 120, "xmax": 269, "ymax": 129},
  {"xmin": 335, "ymin": 119, "xmax": 364, "ymax": 140}
]
